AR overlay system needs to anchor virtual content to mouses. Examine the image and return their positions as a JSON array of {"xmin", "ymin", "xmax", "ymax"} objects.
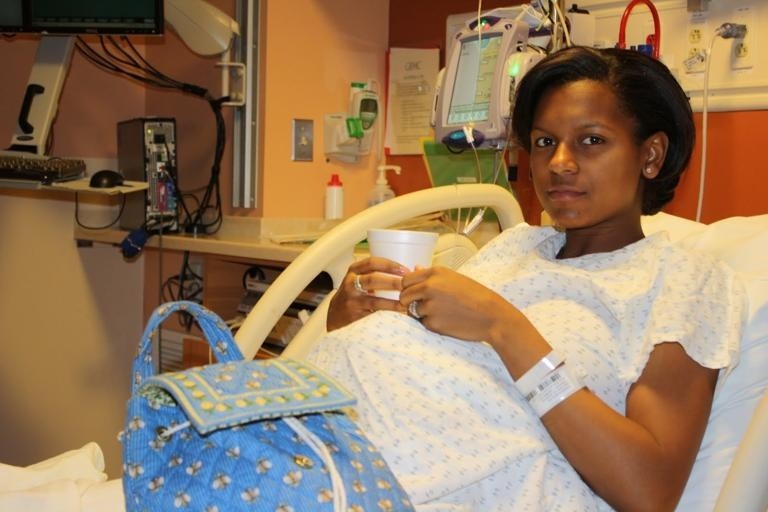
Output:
[{"xmin": 90, "ymin": 170, "xmax": 124, "ymax": 188}]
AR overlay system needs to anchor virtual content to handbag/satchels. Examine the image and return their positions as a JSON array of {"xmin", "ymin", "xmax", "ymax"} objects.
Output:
[{"xmin": 122, "ymin": 300, "xmax": 415, "ymax": 512}]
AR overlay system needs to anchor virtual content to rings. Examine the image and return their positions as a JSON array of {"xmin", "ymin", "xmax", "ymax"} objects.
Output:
[
  {"xmin": 354, "ymin": 272, "xmax": 367, "ymax": 292},
  {"xmin": 408, "ymin": 300, "xmax": 423, "ymax": 322}
]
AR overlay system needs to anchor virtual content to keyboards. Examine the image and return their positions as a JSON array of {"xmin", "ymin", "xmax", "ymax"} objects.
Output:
[{"xmin": 0, "ymin": 154, "xmax": 85, "ymax": 183}]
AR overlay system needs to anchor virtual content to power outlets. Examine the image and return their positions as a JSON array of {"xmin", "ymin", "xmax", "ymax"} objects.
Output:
[
  {"xmin": 684, "ymin": 21, "xmax": 709, "ymax": 72},
  {"xmin": 732, "ymin": 8, "xmax": 754, "ymax": 69}
]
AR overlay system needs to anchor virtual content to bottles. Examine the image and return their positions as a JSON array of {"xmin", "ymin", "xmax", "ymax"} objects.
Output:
[{"xmin": 325, "ymin": 174, "xmax": 344, "ymax": 220}]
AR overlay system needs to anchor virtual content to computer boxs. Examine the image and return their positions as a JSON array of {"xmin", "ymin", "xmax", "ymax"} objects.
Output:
[{"xmin": 116, "ymin": 116, "xmax": 181, "ymax": 234}]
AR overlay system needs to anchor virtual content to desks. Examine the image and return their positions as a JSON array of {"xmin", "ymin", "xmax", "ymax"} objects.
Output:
[{"xmin": 75, "ymin": 214, "xmax": 365, "ymax": 373}]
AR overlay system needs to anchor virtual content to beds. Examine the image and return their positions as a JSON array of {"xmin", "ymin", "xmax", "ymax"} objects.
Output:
[{"xmin": 2, "ymin": 183, "xmax": 768, "ymax": 511}]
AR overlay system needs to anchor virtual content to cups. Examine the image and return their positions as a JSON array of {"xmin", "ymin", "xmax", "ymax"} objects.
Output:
[{"xmin": 366, "ymin": 228, "xmax": 439, "ymax": 302}]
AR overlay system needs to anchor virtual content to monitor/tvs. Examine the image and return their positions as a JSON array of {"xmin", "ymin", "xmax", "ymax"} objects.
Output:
[
  {"xmin": 1, "ymin": 0, "xmax": 164, "ymax": 35},
  {"xmin": 435, "ymin": 21, "xmax": 531, "ymax": 143}
]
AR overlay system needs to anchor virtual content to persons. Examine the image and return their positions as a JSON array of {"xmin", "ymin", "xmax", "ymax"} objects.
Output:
[{"xmin": 295, "ymin": 43, "xmax": 749, "ymax": 512}]
